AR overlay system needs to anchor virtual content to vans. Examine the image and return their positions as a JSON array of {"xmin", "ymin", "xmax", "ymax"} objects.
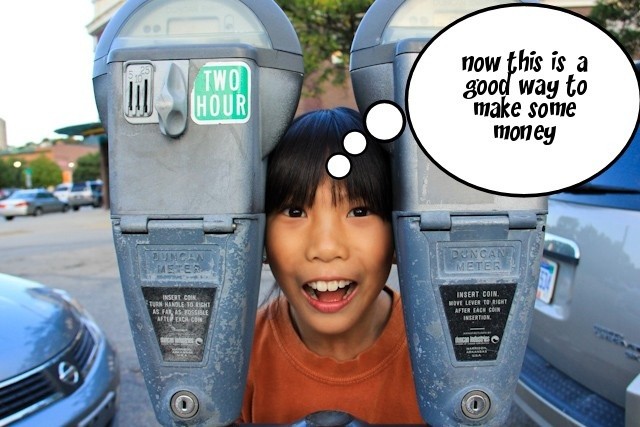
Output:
[
  {"xmin": 52, "ymin": 182, "xmax": 101, "ymax": 211},
  {"xmin": 513, "ymin": 55, "xmax": 640, "ymax": 425}
]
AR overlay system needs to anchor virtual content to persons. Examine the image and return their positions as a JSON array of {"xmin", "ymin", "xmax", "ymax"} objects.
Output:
[{"xmin": 229, "ymin": 106, "xmax": 429, "ymax": 426}]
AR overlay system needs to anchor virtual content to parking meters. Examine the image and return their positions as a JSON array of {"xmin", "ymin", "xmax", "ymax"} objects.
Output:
[
  {"xmin": 92, "ymin": 1, "xmax": 305, "ymax": 427},
  {"xmin": 350, "ymin": 0, "xmax": 551, "ymax": 426}
]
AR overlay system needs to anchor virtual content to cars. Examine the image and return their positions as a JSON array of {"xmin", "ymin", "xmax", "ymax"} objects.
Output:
[
  {"xmin": 0, "ymin": 267, "xmax": 120, "ymax": 427},
  {"xmin": 1, "ymin": 189, "xmax": 70, "ymax": 221},
  {"xmin": 86, "ymin": 179, "xmax": 103, "ymax": 197}
]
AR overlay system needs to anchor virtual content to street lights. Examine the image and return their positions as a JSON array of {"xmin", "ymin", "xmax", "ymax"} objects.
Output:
[
  {"xmin": 12, "ymin": 161, "xmax": 22, "ymax": 187},
  {"xmin": 60, "ymin": 162, "xmax": 75, "ymax": 183}
]
